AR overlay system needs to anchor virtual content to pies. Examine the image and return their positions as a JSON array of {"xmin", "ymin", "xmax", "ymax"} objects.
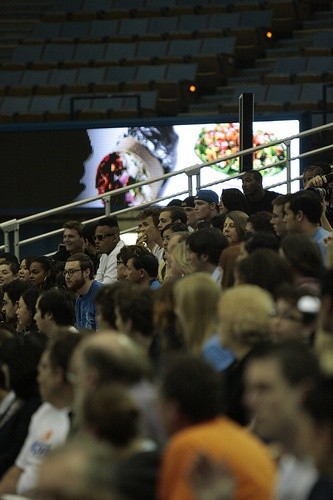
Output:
[{"xmin": 94, "ymin": 140, "xmax": 166, "ymax": 207}]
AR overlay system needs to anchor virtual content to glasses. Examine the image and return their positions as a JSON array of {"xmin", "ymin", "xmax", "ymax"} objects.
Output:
[
  {"xmin": 93, "ymin": 234, "xmax": 113, "ymax": 241},
  {"xmin": 63, "ymin": 270, "xmax": 82, "ymax": 276},
  {"xmin": 117, "ymin": 260, "xmax": 124, "ymax": 265}
]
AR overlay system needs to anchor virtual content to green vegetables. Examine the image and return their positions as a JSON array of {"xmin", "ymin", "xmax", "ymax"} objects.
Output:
[{"xmin": 194, "ymin": 129, "xmax": 287, "ymax": 178}]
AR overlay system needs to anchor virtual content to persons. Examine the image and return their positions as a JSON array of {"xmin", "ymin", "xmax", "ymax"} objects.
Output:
[{"xmin": 0, "ymin": 165, "xmax": 332, "ymax": 500}]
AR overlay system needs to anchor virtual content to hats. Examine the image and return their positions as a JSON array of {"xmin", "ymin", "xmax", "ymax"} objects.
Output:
[
  {"xmin": 194, "ymin": 190, "xmax": 220, "ymax": 214},
  {"xmin": 181, "ymin": 196, "xmax": 195, "ymax": 207}
]
